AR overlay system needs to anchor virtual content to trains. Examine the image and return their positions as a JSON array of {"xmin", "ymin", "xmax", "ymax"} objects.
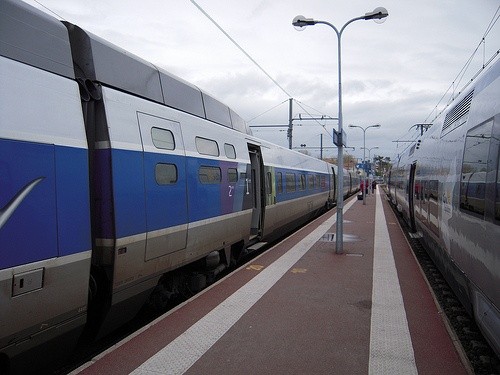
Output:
[
  {"xmin": 0, "ymin": 0, "xmax": 362, "ymax": 363},
  {"xmin": 383, "ymin": 57, "xmax": 500, "ymax": 360}
]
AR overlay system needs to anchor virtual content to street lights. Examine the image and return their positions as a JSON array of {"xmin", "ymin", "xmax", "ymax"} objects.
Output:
[{"xmin": 293, "ymin": 6, "xmax": 391, "ymax": 256}]
